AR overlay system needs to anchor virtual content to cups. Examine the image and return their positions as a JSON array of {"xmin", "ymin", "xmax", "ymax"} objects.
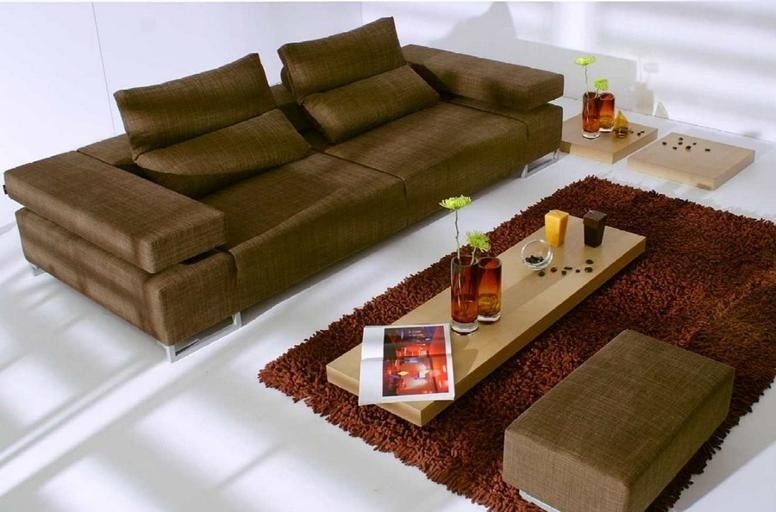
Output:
[{"xmin": 614, "ymin": 127, "xmax": 629, "ymax": 138}]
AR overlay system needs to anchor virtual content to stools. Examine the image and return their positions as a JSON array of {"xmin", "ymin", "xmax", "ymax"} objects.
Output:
[{"xmin": 502, "ymin": 329, "xmax": 737, "ymax": 512}]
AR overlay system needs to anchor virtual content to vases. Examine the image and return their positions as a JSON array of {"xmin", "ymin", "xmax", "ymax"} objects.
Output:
[
  {"xmin": 582, "ymin": 92, "xmax": 614, "ymax": 139},
  {"xmin": 450, "ymin": 256, "xmax": 502, "ymax": 335}
]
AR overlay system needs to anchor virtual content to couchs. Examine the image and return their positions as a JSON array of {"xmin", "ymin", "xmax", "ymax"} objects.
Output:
[{"xmin": 5, "ymin": 45, "xmax": 563, "ymax": 364}]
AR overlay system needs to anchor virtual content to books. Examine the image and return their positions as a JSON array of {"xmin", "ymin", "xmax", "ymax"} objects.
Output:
[{"xmin": 357, "ymin": 323, "xmax": 457, "ymax": 404}]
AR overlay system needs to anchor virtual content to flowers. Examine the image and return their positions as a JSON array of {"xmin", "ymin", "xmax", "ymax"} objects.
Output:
[
  {"xmin": 439, "ymin": 195, "xmax": 493, "ymax": 320},
  {"xmin": 575, "ymin": 56, "xmax": 609, "ymax": 132}
]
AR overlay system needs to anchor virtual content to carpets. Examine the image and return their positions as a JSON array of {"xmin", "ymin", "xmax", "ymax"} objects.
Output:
[{"xmin": 258, "ymin": 175, "xmax": 776, "ymax": 512}]
